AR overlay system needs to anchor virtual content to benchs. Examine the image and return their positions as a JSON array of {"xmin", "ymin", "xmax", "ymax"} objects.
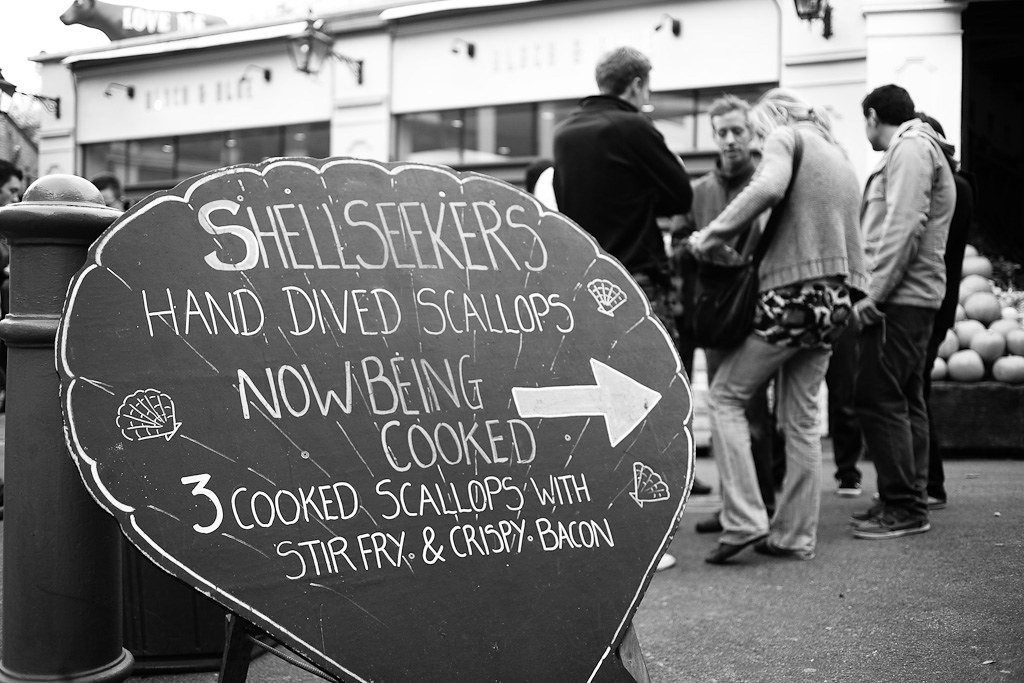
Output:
[{"xmin": 929, "ymin": 381, "xmax": 1024, "ymax": 448}]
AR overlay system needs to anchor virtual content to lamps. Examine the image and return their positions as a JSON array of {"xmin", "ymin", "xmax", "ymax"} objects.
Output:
[
  {"xmin": 452, "ymin": 37, "xmax": 475, "ymax": 56},
  {"xmin": 103, "ymin": 82, "xmax": 133, "ymax": 98},
  {"xmin": 654, "ymin": 13, "xmax": 681, "ymax": 37},
  {"xmin": 794, "ymin": 0, "xmax": 834, "ymax": 40},
  {"xmin": 239, "ymin": 63, "xmax": 270, "ymax": 82}
]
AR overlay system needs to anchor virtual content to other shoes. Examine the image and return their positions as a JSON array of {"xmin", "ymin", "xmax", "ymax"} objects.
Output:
[
  {"xmin": 848, "ymin": 502, "xmax": 882, "ymax": 521},
  {"xmin": 695, "ymin": 509, "xmax": 725, "ymax": 534},
  {"xmin": 926, "ymin": 495, "xmax": 945, "ymax": 509},
  {"xmin": 656, "ymin": 552, "xmax": 677, "ymax": 569},
  {"xmin": 849, "ymin": 508, "xmax": 932, "ymax": 539},
  {"xmin": 836, "ymin": 476, "xmax": 861, "ymax": 495},
  {"xmin": 690, "ymin": 477, "xmax": 712, "ymax": 494},
  {"xmin": 706, "ymin": 533, "xmax": 767, "ymax": 563},
  {"xmin": 754, "ymin": 538, "xmax": 798, "ymax": 556}
]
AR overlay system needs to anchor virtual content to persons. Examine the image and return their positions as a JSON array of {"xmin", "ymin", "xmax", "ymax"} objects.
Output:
[
  {"xmin": 915, "ymin": 111, "xmax": 966, "ymax": 508},
  {"xmin": 527, "ymin": 158, "xmax": 554, "ymax": 195},
  {"xmin": 673, "ymin": 95, "xmax": 786, "ymax": 534},
  {"xmin": 0, "ymin": 161, "xmax": 31, "ymax": 517},
  {"xmin": 684, "ymin": 88, "xmax": 867, "ymax": 563},
  {"xmin": 90, "ymin": 171, "xmax": 124, "ymax": 212},
  {"xmin": 551, "ymin": 42, "xmax": 694, "ymax": 358},
  {"xmin": 844, "ymin": 85, "xmax": 955, "ymax": 539}
]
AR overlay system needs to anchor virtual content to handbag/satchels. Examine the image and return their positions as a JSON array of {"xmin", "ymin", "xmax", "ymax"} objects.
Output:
[{"xmin": 682, "ymin": 249, "xmax": 760, "ymax": 351}]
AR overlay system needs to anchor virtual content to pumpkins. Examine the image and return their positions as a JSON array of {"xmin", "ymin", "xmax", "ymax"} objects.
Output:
[{"xmin": 927, "ymin": 246, "xmax": 1024, "ymax": 383}]
